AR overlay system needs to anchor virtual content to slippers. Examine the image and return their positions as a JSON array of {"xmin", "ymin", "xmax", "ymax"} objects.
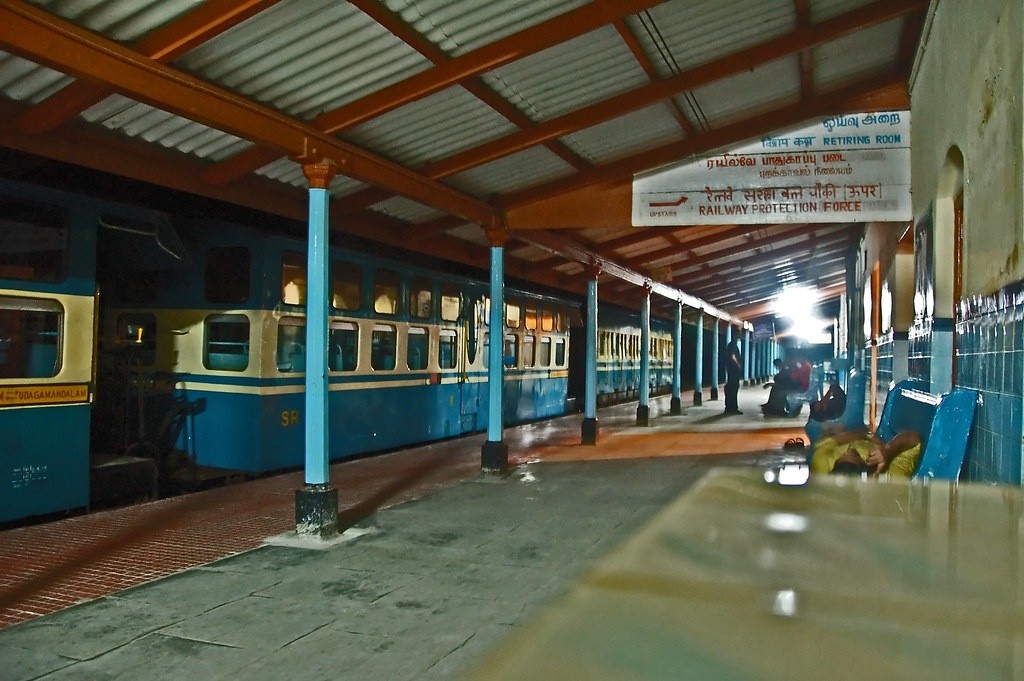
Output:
[
  {"xmin": 783, "ymin": 439, "xmax": 796, "ymax": 451},
  {"xmin": 796, "ymin": 437, "xmax": 804, "ymax": 451}
]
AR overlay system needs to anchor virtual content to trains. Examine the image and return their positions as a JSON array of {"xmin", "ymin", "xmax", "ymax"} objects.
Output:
[{"xmin": 0, "ymin": 190, "xmax": 673, "ymax": 532}]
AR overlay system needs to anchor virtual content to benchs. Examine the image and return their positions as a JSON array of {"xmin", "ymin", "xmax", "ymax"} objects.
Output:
[{"xmin": 787, "ymin": 366, "xmax": 977, "ymax": 484}]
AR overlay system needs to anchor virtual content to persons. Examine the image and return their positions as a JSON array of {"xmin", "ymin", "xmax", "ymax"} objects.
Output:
[
  {"xmin": 810, "ymin": 384, "xmax": 846, "ymax": 422},
  {"xmin": 760, "ymin": 358, "xmax": 811, "ymax": 417},
  {"xmin": 724, "ymin": 324, "xmax": 743, "ymax": 415},
  {"xmin": 812, "ymin": 423, "xmax": 922, "ymax": 478}
]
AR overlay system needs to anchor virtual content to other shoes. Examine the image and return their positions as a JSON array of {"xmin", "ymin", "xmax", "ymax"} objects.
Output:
[{"xmin": 724, "ymin": 409, "xmax": 743, "ymax": 415}]
